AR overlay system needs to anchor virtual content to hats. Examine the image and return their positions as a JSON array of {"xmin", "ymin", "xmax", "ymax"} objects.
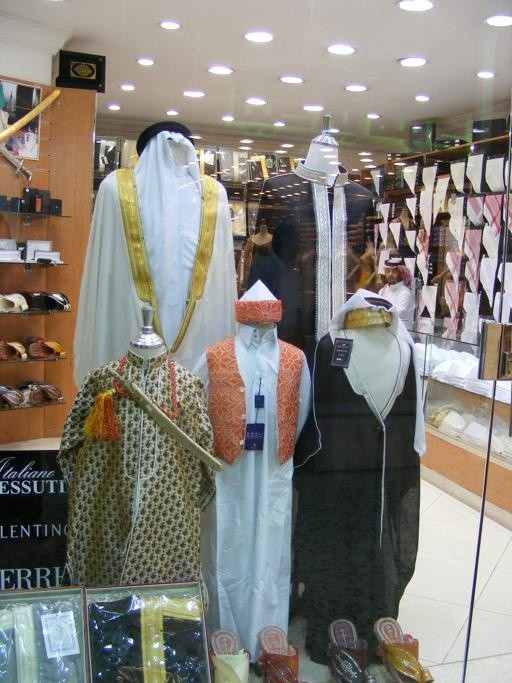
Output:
[{"xmin": 137, "ymin": 118, "xmax": 195, "ymax": 156}]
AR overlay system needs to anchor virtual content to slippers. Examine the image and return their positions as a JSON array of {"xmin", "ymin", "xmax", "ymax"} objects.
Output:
[
  {"xmin": 0, "ymin": 383, "xmax": 65, "ymax": 409},
  {"xmin": 0, "ymin": 339, "xmax": 68, "ymax": 362},
  {"xmin": 325, "ymin": 616, "xmax": 369, "ymax": 683},
  {"xmin": 256, "ymin": 625, "xmax": 299, "ymax": 683},
  {"xmin": 208, "ymin": 628, "xmax": 252, "ymax": 683},
  {"xmin": 372, "ymin": 617, "xmax": 434, "ymax": 683},
  {"xmin": 0, "ymin": 290, "xmax": 72, "ymax": 313}
]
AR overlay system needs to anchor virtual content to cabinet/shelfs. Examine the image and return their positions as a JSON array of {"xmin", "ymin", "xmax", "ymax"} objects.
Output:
[
  {"xmin": 404, "ymin": 319, "xmax": 512, "ymax": 532},
  {"xmin": 0, "ymin": 209, "xmax": 71, "ymax": 412}
]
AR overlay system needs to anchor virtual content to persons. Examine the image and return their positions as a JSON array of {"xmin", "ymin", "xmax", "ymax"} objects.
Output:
[
  {"xmin": 376, "ymin": 256, "xmax": 414, "ymax": 318},
  {"xmin": 297, "ymin": 293, "xmax": 421, "ymax": 660},
  {"xmin": 72, "ymin": 125, "xmax": 231, "ymax": 376},
  {"xmin": 195, "ymin": 277, "xmax": 316, "ymax": 663},
  {"xmin": 250, "ymin": 125, "xmax": 376, "ymax": 365},
  {"xmin": 51, "ymin": 322, "xmax": 208, "ymax": 589}
]
